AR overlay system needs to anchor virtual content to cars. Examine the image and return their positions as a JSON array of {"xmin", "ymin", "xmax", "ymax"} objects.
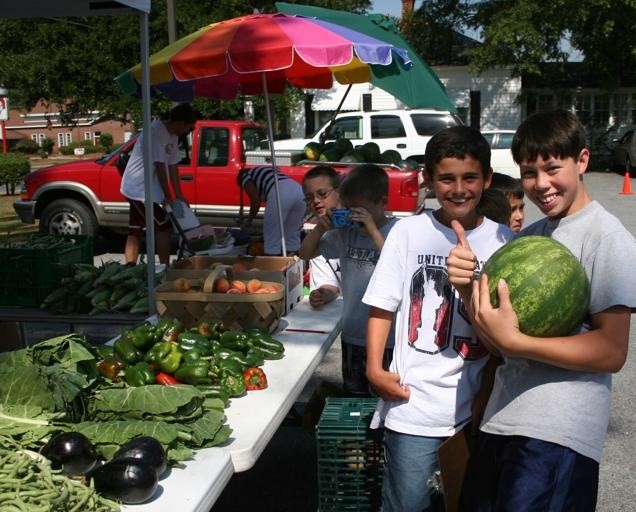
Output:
[
  {"xmin": 471, "ymin": 127, "xmax": 527, "ymax": 179},
  {"xmin": 607, "ymin": 127, "xmax": 635, "ymax": 174}
]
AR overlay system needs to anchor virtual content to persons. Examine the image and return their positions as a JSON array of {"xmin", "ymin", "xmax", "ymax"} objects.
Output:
[
  {"xmin": 490, "ymin": 172, "xmax": 526, "ymax": 233},
  {"xmin": 447, "ymin": 109, "xmax": 636, "ymax": 512},
  {"xmin": 298, "ymin": 164, "xmax": 401, "ymax": 398},
  {"xmin": 301, "ymin": 166, "xmax": 345, "ymax": 307},
  {"xmin": 120, "ymin": 102, "xmax": 196, "ymax": 265},
  {"xmin": 362, "ymin": 126, "xmax": 517, "ymax": 512},
  {"xmin": 475, "ymin": 187, "xmax": 511, "ymax": 228},
  {"xmin": 236, "ymin": 166, "xmax": 307, "ymax": 255}
]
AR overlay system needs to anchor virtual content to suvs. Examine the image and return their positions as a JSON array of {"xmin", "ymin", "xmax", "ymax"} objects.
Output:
[{"xmin": 252, "ymin": 104, "xmax": 467, "ymax": 171}]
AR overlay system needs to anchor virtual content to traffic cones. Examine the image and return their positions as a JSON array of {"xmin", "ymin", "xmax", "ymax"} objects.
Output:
[{"xmin": 618, "ymin": 171, "xmax": 635, "ymax": 195}]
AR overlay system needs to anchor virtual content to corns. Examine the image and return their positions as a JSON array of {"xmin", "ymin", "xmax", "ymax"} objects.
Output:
[{"xmin": 40, "ymin": 260, "xmax": 164, "ymax": 315}]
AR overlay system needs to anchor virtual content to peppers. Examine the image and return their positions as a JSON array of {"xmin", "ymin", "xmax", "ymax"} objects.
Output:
[{"xmin": 95, "ymin": 316, "xmax": 285, "ymax": 402}]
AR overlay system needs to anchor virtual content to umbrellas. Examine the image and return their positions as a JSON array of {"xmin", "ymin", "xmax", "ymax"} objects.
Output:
[
  {"xmin": 113, "ymin": 8, "xmax": 414, "ymax": 257},
  {"xmin": 274, "ymin": 2, "xmax": 459, "ymax": 145}
]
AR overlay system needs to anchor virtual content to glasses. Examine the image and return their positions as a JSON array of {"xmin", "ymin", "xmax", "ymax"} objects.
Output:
[{"xmin": 302, "ymin": 188, "xmax": 339, "ymax": 207}]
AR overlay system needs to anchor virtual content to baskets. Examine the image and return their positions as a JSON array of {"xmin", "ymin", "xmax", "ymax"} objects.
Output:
[{"xmin": 152, "ymin": 265, "xmax": 286, "ymax": 335}]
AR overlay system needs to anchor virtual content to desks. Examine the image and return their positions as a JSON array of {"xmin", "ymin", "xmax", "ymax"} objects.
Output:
[
  {"xmin": 1, "ymin": 290, "xmax": 349, "ymax": 346},
  {"xmin": 83, "ymin": 446, "xmax": 237, "ymax": 512},
  {"xmin": 101, "ymin": 320, "xmax": 334, "ymax": 482}
]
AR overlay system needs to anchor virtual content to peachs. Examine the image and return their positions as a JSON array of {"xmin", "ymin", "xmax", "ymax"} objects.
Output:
[{"xmin": 171, "ymin": 261, "xmax": 288, "ymax": 295}]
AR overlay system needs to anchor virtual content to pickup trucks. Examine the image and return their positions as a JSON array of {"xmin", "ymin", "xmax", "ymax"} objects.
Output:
[{"xmin": 10, "ymin": 117, "xmax": 428, "ymax": 241}]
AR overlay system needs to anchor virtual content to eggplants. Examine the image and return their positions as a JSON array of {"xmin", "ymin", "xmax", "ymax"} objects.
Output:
[
  {"xmin": 114, "ymin": 435, "xmax": 167, "ymax": 476},
  {"xmin": 44, "ymin": 432, "xmax": 97, "ymax": 475},
  {"xmin": 91, "ymin": 458, "xmax": 158, "ymax": 504}
]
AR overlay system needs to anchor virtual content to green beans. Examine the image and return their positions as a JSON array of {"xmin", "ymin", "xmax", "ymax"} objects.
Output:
[
  {"xmin": 4, "ymin": 232, "xmax": 77, "ymax": 302},
  {"xmin": 0, "ymin": 448, "xmax": 120, "ymax": 512}
]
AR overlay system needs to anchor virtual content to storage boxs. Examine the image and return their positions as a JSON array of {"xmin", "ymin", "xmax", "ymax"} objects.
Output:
[
  {"xmin": 163, "ymin": 252, "xmax": 306, "ymax": 320},
  {"xmin": 0, "ymin": 228, "xmax": 96, "ymax": 310},
  {"xmin": 244, "ymin": 150, "xmax": 304, "ymax": 166}
]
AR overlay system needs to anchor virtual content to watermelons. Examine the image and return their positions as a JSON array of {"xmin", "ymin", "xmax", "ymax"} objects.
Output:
[
  {"xmin": 481, "ymin": 236, "xmax": 590, "ymax": 337},
  {"xmin": 290, "ymin": 137, "xmax": 419, "ymax": 171},
  {"xmin": 189, "ymin": 234, "xmax": 213, "ymax": 250}
]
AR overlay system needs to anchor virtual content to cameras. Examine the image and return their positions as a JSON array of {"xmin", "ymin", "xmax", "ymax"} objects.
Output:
[{"xmin": 331, "ymin": 209, "xmax": 362, "ymax": 228}]
